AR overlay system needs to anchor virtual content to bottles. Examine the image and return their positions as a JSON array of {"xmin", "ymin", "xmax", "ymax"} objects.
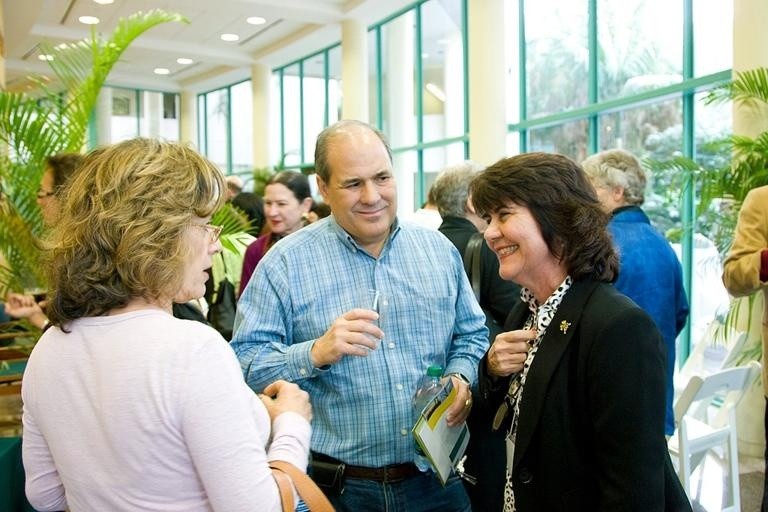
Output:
[{"xmin": 412, "ymin": 364, "xmax": 447, "ymax": 476}]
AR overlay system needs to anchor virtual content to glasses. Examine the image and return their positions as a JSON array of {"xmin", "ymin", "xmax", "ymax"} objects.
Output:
[
  {"xmin": 35, "ymin": 187, "xmax": 55, "ymax": 199},
  {"xmin": 190, "ymin": 219, "xmax": 224, "ymax": 244}
]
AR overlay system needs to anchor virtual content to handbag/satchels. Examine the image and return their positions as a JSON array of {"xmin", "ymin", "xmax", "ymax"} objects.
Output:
[
  {"xmin": 471, "ymin": 308, "xmax": 508, "ymax": 402},
  {"xmin": 307, "ymin": 458, "xmax": 346, "ymax": 499}
]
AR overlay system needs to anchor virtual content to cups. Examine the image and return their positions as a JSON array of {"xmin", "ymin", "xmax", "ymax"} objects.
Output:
[{"xmin": 350, "ymin": 286, "xmax": 392, "ymax": 356}]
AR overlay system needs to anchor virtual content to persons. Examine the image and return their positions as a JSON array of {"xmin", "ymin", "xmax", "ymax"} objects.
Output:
[
  {"xmin": 722, "ymin": 186, "xmax": 768, "ymax": 512},
  {"xmin": 434, "ymin": 168, "xmax": 521, "ymax": 512},
  {"xmin": 21, "ymin": 138, "xmax": 314, "ymax": 512},
  {"xmin": 581, "ymin": 150, "xmax": 690, "ymax": 445},
  {"xmin": 228, "ymin": 120, "xmax": 490, "ymax": 512},
  {"xmin": 469, "ymin": 153, "xmax": 694, "ymax": 512}
]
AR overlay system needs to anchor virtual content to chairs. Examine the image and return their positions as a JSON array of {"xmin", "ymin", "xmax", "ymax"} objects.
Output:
[
  {"xmin": 657, "ymin": 359, "xmax": 763, "ymax": 511},
  {"xmin": 665, "ymin": 320, "xmax": 748, "ymax": 419}
]
